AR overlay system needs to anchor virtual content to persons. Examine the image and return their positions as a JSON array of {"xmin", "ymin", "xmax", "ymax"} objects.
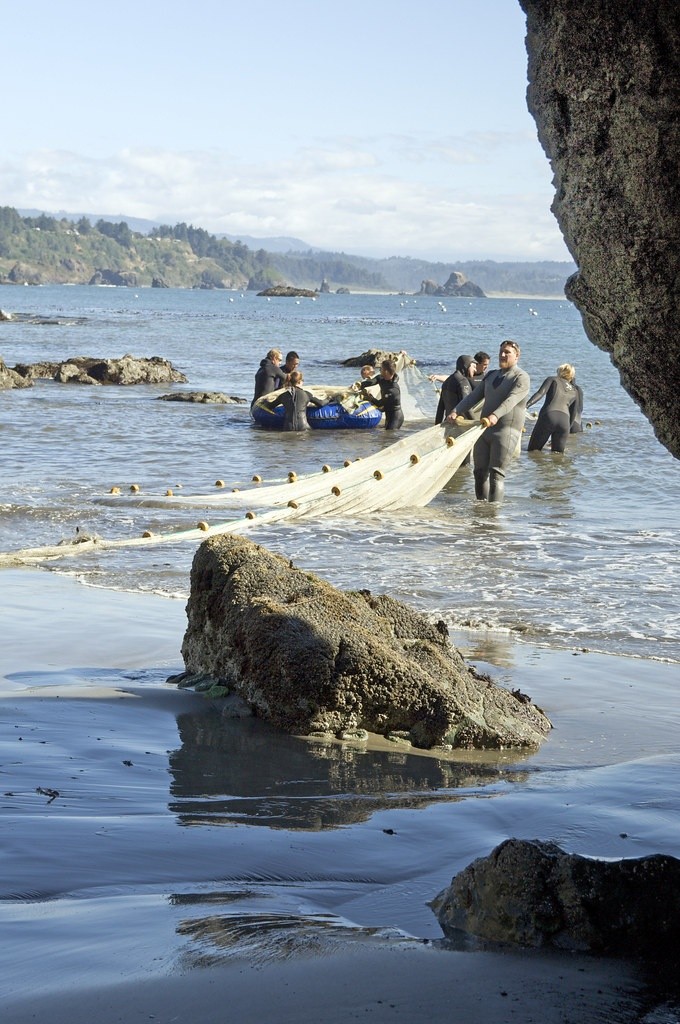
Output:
[
  {"xmin": 526, "ymin": 363, "xmax": 580, "ymax": 452},
  {"xmin": 569, "ymin": 379, "xmax": 583, "ymax": 433},
  {"xmin": 446, "ymin": 340, "xmax": 530, "ymax": 502},
  {"xmin": 428, "ymin": 351, "xmax": 490, "ymax": 425},
  {"xmin": 360, "ymin": 357, "xmax": 404, "ymax": 430},
  {"xmin": 251, "ymin": 348, "xmax": 334, "ymax": 431}
]
